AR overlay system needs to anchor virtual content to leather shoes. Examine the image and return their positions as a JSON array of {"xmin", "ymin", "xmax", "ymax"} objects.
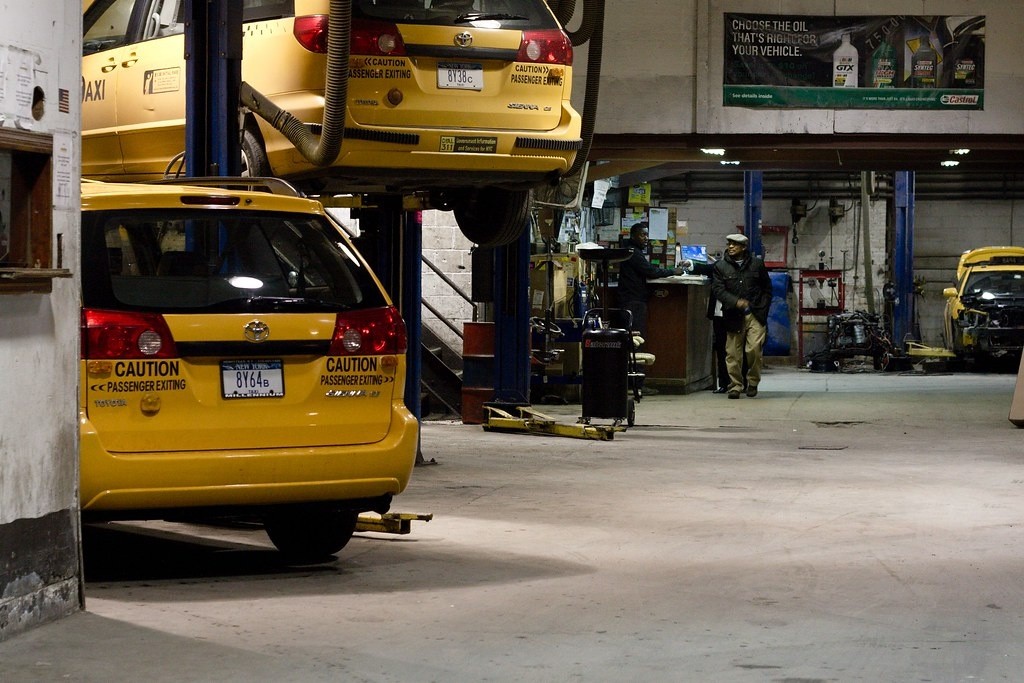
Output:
[
  {"xmin": 713, "ymin": 387, "xmax": 728, "ymax": 393},
  {"xmin": 728, "ymin": 385, "xmax": 758, "ymax": 399}
]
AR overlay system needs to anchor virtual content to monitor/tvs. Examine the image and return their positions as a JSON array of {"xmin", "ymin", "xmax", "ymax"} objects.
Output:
[{"xmin": 680, "ymin": 243, "xmax": 707, "ymax": 266}]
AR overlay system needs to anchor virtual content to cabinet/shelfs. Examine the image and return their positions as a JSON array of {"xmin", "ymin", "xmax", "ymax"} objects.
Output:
[
  {"xmin": 531, "ymin": 252, "xmax": 582, "ymax": 401},
  {"xmin": 798, "ymin": 269, "xmax": 846, "ymax": 367}
]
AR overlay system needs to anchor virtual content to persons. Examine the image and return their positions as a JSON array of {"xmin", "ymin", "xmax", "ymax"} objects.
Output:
[
  {"xmin": 710, "ymin": 233, "xmax": 772, "ymax": 398},
  {"xmin": 616, "ymin": 222, "xmax": 685, "ymax": 396},
  {"xmin": 675, "ymin": 249, "xmax": 749, "ymax": 394}
]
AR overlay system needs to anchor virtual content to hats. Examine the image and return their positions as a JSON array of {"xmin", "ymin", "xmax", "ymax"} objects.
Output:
[{"xmin": 726, "ymin": 233, "xmax": 749, "ymax": 246}]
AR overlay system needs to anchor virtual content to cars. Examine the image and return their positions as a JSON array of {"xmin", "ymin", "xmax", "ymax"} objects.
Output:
[{"xmin": 942, "ymin": 245, "xmax": 1024, "ymax": 358}]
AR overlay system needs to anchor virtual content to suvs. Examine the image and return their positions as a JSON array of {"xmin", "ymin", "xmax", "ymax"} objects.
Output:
[
  {"xmin": 80, "ymin": 172, "xmax": 418, "ymax": 563},
  {"xmin": 81, "ymin": 0, "xmax": 585, "ymax": 246}
]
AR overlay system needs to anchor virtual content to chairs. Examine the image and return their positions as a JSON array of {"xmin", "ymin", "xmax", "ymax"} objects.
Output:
[{"xmin": 157, "ymin": 250, "xmax": 207, "ymax": 277}]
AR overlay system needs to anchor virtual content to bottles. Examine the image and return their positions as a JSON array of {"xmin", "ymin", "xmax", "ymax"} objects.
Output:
[
  {"xmin": 910, "ymin": 35, "xmax": 938, "ymax": 89},
  {"xmin": 832, "ymin": 32, "xmax": 857, "ymax": 89},
  {"xmin": 942, "ymin": 32, "xmax": 984, "ymax": 88},
  {"xmin": 868, "ymin": 33, "xmax": 897, "ymax": 89}
]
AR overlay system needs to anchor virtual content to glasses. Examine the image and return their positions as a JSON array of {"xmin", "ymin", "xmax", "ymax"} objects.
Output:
[
  {"xmin": 632, "ymin": 232, "xmax": 649, "ymax": 237},
  {"xmin": 726, "ymin": 241, "xmax": 744, "ymax": 246}
]
